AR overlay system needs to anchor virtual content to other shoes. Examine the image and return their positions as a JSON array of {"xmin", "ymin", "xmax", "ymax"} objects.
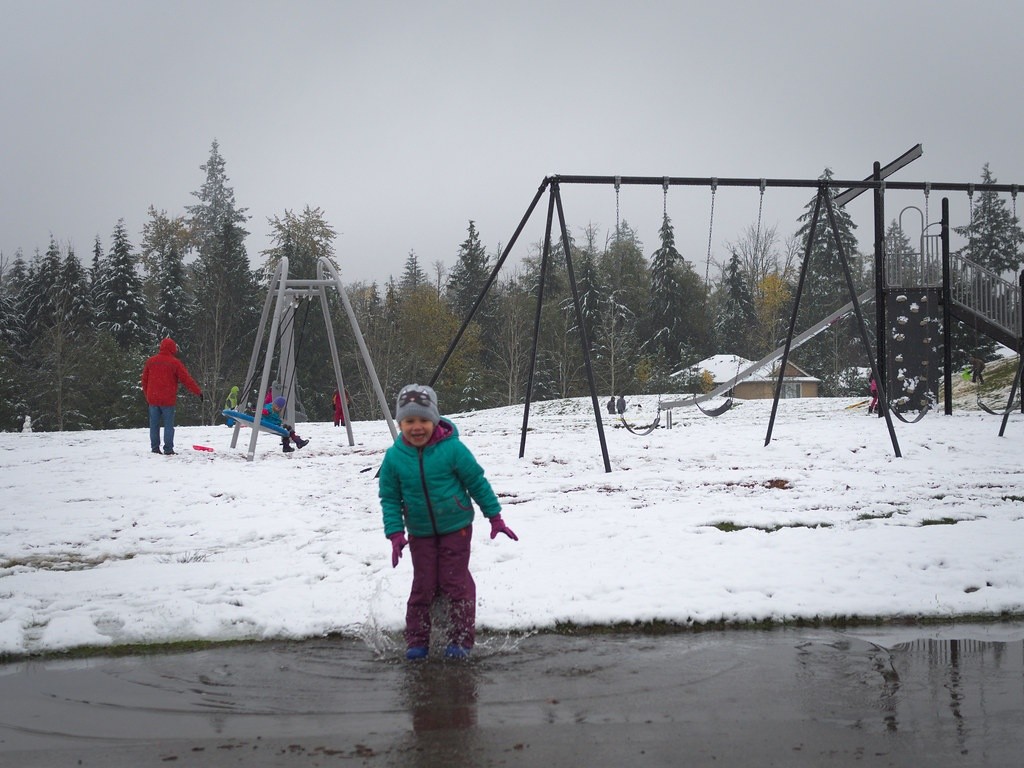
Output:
[
  {"xmin": 445, "ymin": 642, "xmax": 472, "ymax": 658},
  {"xmin": 164, "ymin": 449, "xmax": 178, "ymax": 455},
  {"xmin": 151, "ymin": 450, "xmax": 163, "ymax": 455},
  {"xmin": 406, "ymin": 646, "xmax": 429, "ymax": 659}
]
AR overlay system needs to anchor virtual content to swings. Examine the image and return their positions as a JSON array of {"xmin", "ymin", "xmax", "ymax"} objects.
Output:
[
  {"xmin": 613, "ymin": 184, "xmax": 669, "ymax": 437},
  {"xmin": 967, "ymin": 194, "xmax": 1021, "ymax": 417},
  {"xmin": 692, "ymin": 190, "xmax": 763, "ymax": 417},
  {"xmin": 879, "ymin": 189, "xmax": 932, "ymax": 425},
  {"xmin": 222, "ymin": 295, "xmax": 313, "ymax": 439}
]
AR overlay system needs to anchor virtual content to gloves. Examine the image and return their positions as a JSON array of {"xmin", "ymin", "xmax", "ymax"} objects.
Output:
[
  {"xmin": 389, "ymin": 531, "xmax": 408, "ymax": 568},
  {"xmin": 489, "ymin": 514, "xmax": 519, "ymax": 541},
  {"xmin": 198, "ymin": 392, "xmax": 204, "ymax": 402}
]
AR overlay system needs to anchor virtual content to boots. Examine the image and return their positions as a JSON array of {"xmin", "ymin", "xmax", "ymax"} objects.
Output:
[
  {"xmin": 294, "ymin": 436, "xmax": 309, "ymax": 449},
  {"xmin": 282, "ymin": 439, "xmax": 294, "ymax": 452}
]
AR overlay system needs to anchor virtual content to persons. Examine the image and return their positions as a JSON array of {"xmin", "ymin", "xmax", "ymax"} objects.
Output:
[
  {"xmin": 607, "ymin": 396, "xmax": 615, "ymax": 414},
  {"xmin": 225, "ymin": 385, "xmax": 239, "ymax": 427},
  {"xmin": 377, "ymin": 383, "xmax": 518, "ymax": 660},
  {"xmin": 263, "ymin": 396, "xmax": 309, "ymax": 452},
  {"xmin": 868, "ymin": 373, "xmax": 879, "ymax": 413},
  {"xmin": 617, "ymin": 394, "xmax": 626, "ymax": 414},
  {"xmin": 967, "ymin": 357, "xmax": 985, "ymax": 384},
  {"xmin": 262, "ymin": 386, "xmax": 272, "ymax": 415},
  {"xmin": 333, "ymin": 388, "xmax": 350, "ymax": 426},
  {"xmin": 142, "ymin": 338, "xmax": 203, "ymax": 455}
]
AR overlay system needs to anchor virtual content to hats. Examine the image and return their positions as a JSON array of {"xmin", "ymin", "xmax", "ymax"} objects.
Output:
[
  {"xmin": 396, "ymin": 383, "xmax": 441, "ymax": 426},
  {"xmin": 274, "ymin": 397, "xmax": 285, "ymax": 407}
]
